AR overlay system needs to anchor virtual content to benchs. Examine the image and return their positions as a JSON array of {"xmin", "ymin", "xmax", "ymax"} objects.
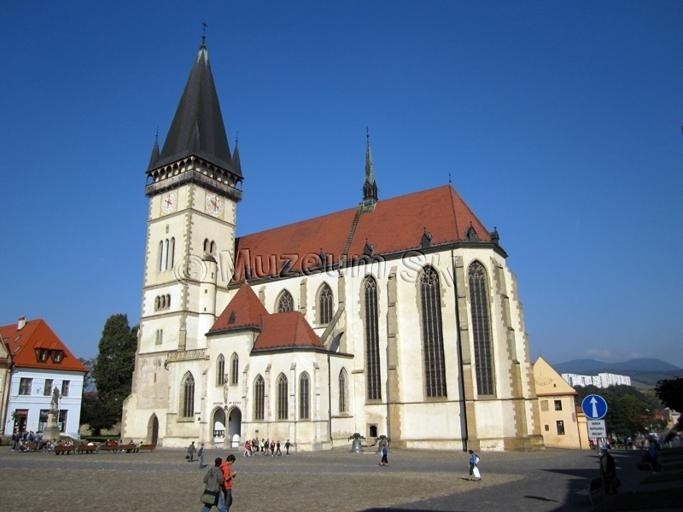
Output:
[{"xmin": 54, "ymin": 444, "xmax": 155, "ymax": 455}]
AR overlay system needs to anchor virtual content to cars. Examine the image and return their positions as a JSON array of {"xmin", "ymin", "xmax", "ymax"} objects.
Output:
[{"xmin": 631, "ymin": 431, "xmax": 672, "ymax": 450}]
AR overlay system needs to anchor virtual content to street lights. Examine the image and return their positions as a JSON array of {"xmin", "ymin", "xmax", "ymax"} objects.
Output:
[{"xmin": 0, "ymin": 362, "xmax": 19, "ymax": 428}]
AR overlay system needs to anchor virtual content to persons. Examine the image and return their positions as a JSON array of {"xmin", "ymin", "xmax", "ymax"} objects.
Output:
[
  {"xmin": 379, "ymin": 444, "xmax": 388, "ymax": 466},
  {"xmin": 242, "ymin": 436, "xmax": 293, "ymax": 458},
  {"xmin": 598, "ymin": 448, "xmax": 618, "ymax": 494},
  {"xmin": 200, "ymin": 457, "xmax": 227, "ymax": 512},
  {"xmin": 588, "ymin": 432, "xmax": 661, "ymax": 478},
  {"xmin": 380, "ymin": 444, "xmax": 384, "ymax": 465},
  {"xmin": 218, "ymin": 454, "xmax": 236, "ymax": 509},
  {"xmin": 197, "ymin": 442, "xmax": 206, "ymax": 469},
  {"xmin": 186, "ymin": 441, "xmax": 197, "ymax": 461},
  {"xmin": 465, "ymin": 449, "xmax": 477, "ymax": 480},
  {"xmin": 10, "ymin": 427, "xmax": 143, "ymax": 455},
  {"xmin": 473, "ymin": 452, "xmax": 481, "ymax": 480}
]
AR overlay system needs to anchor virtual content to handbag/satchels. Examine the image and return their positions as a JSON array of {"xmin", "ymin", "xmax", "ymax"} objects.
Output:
[{"xmin": 198, "ymin": 488, "xmax": 219, "ymax": 505}]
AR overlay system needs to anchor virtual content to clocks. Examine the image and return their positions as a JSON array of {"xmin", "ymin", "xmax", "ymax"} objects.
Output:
[
  {"xmin": 206, "ymin": 192, "xmax": 225, "ymax": 217},
  {"xmin": 161, "ymin": 192, "xmax": 176, "ymax": 213}
]
